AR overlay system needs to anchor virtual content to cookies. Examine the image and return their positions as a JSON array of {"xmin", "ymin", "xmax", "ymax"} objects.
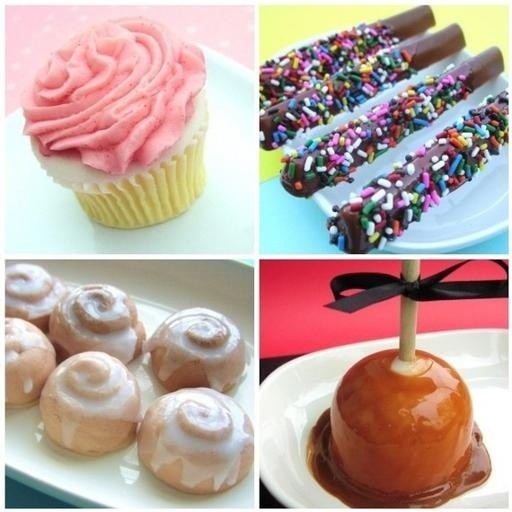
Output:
[{"xmin": 259, "ymin": 3, "xmax": 510, "ymax": 254}]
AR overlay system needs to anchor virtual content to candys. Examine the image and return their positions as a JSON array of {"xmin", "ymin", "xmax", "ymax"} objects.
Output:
[{"xmin": 328, "ymin": 260, "xmax": 472, "ymax": 494}]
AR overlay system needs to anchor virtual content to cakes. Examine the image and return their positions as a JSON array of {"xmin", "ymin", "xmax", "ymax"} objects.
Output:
[
  {"xmin": 7, "ymin": 317, "xmax": 57, "ymax": 406},
  {"xmin": 49, "ymin": 284, "xmax": 147, "ymax": 364},
  {"xmin": 5, "ymin": 263, "xmax": 68, "ymax": 334},
  {"xmin": 148, "ymin": 308, "xmax": 253, "ymax": 391},
  {"xmin": 39, "ymin": 350, "xmax": 144, "ymax": 455},
  {"xmin": 22, "ymin": 20, "xmax": 210, "ymax": 228},
  {"xmin": 138, "ymin": 386, "xmax": 253, "ymax": 493}
]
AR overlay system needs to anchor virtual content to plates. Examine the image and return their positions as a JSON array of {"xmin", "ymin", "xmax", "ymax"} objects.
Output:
[
  {"xmin": 4, "ymin": 41, "xmax": 254, "ymax": 255},
  {"xmin": 5, "ymin": 280, "xmax": 253, "ymax": 509},
  {"xmin": 283, "ymin": 30, "xmax": 508, "ymax": 253},
  {"xmin": 259, "ymin": 328, "xmax": 509, "ymax": 508}
]
